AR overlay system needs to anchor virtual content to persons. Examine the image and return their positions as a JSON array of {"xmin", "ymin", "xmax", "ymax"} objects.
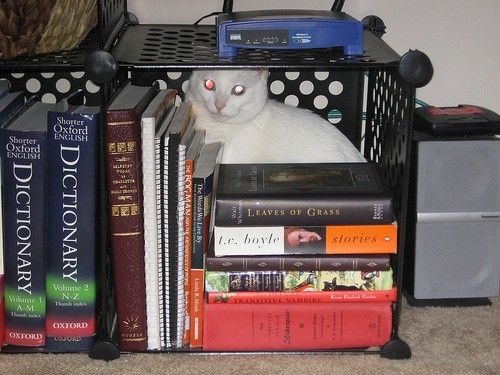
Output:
[{"xmin": 284, "ymin": 227, "xmax": 322, "ymax": 252}]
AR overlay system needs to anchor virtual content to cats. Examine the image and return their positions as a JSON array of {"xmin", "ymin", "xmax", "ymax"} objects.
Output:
[{"xmin": 186, "ymin": 70, "xmax": 368, "ymax": 165}]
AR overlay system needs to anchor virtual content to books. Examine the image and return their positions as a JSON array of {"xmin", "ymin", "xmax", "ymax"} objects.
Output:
[{"xmin": 0, "ymin": 79, "xmax": 397, "ymax": 353}]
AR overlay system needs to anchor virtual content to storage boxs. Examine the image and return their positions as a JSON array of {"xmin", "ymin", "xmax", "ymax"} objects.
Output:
[
  {"xmin": 1, "ymin": 0, "xmax": 432, "ymax": 357},
  {"xmin": 411, "ymin": 135, "xmax": 499, "ymax": 299}
]
